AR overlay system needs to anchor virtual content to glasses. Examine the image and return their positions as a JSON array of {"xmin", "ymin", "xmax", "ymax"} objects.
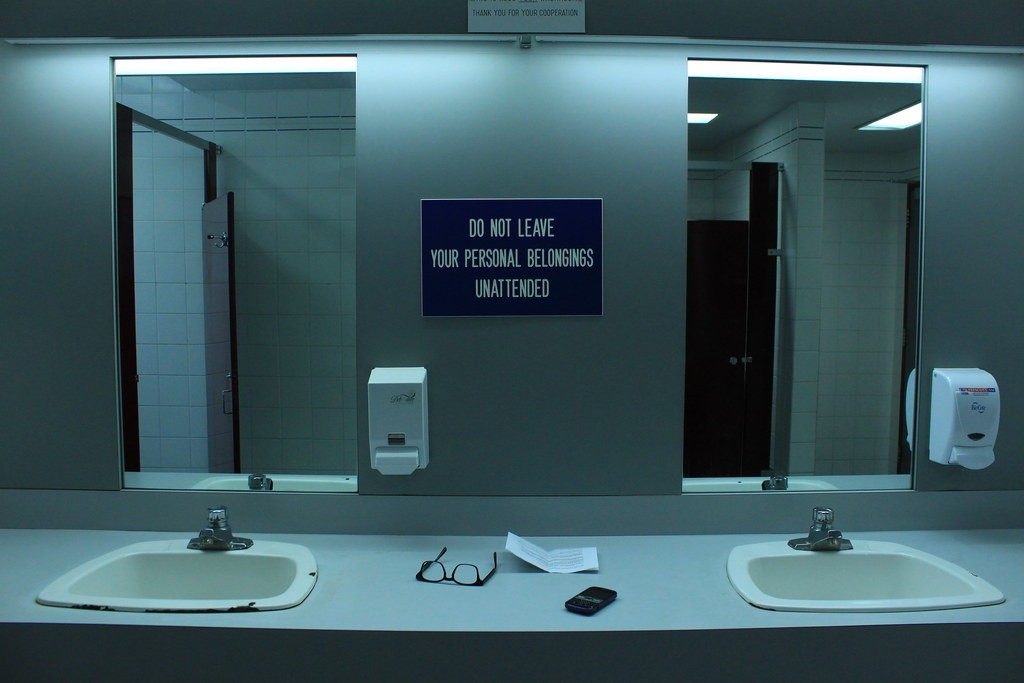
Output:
[{"xmin": 416, "ymin": 547, "xmax": 497, "ymax": 586}]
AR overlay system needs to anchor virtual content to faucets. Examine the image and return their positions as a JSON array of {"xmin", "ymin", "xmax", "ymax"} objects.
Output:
[
  {"xmin": 787, "ymin": 507, "xmax": 853, "ymax": 551},
  {"xmin": 184, "ymin": 506, "xmax": 254, "ymax": 551},
  {"xmin": 762, "ymin": 474, "xmax": 788, "ymax": 491},
  {"xmin": 247, "ymin": 474, "xmax": 273, "ymax": 490}
]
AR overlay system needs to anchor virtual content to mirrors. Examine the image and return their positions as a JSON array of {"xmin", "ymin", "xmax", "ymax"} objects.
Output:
[
  {"xmin": 115, "ymin": 57, "xmax": 359, "ymax": 492},
  {"xmin": 682, "ymin": 57, "xmax": 926, "ymax": 492}
]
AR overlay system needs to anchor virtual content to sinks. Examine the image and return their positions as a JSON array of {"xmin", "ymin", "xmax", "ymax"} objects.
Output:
[
  {"xmin": 682, "ymin": 477, "xmax": 838, "ymax": 492},
  {"xmin": 35, "ymin": 538, "xmax": 320, "ymax": 614},
  {"xmin": 726, "ymin": 538, "xmax": 1008, "ymax": 612},
  {"xmin": 191, "ymin": 476, "xmax": 358, "ymax": 493}
]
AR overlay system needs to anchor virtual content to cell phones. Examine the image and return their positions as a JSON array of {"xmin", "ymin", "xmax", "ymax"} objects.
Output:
[{"xmin": 564, "ymin": 586, "xmax": 617, "ymax": 614}]
